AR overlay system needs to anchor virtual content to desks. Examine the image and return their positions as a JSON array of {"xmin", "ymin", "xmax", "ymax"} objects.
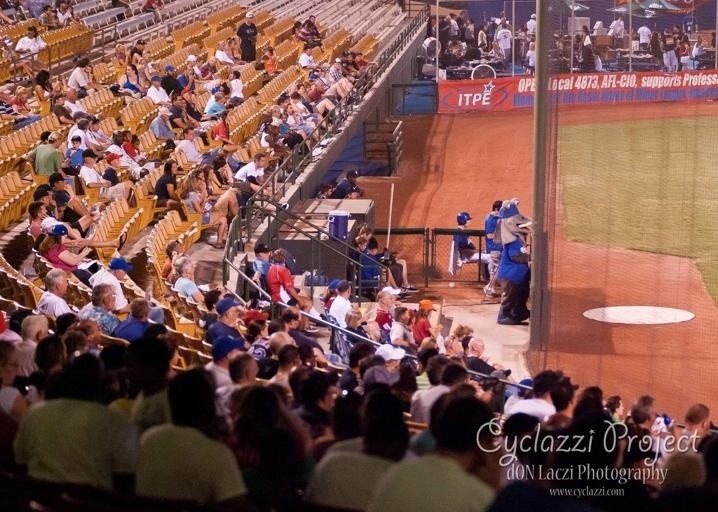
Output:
[{"xmin": 427, "ymin": 22, "xmax": 718, "ymax": 82}]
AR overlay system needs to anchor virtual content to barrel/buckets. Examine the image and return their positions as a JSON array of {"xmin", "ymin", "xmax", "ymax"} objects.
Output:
[{"xmin": 328, "ymin": 210, "xmax": 350, "ymax": 241}]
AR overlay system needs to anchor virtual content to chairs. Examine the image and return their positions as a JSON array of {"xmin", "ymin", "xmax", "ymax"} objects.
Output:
[{"xmin": 449, "ymin": 240, "xmax": 487, "ymax": 288}]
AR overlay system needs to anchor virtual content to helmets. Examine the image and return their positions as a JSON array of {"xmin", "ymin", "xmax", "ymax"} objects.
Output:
[{"xmin": 457, "ymin": 212, "xmax": 470, "ymax": 224}]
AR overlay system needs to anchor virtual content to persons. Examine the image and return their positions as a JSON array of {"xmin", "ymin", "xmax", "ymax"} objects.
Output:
[
  {"xmin": 482, "ymin": 201, "xmax": 505, "ymax": 298},
  {"xmin": 430, "ymin": 11, "xmax": 717, "ymax": 74},
  {"xmin": 453, "ymin": 212, "xmax": 497, "ymax": 298}
]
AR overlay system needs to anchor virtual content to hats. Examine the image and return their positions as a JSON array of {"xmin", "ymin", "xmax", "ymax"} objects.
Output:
[
  {"xmin": 26, "ymin": 12, "xmax": 362, "ymax": 244},
  {"xmin": 111, "ymin": 257, "xmax": 133, "ymax": 272},
  {"xmin": 216, "ymin": 298, "xmax": 239, "ymax": 314}
]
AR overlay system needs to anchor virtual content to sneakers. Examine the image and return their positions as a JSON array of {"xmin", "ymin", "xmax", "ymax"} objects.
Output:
[
  {"xmin": 483, "ymin": 285, "xmax": 499, "ymax": 298},
  {"xmin": 395, "ymin": 283, "xmax": 420, "ymax": 302}
]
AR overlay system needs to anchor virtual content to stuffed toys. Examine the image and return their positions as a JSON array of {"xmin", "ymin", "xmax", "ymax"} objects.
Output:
[{"xmin": 495, "ymin": 197, "xmax": 536, "ymax": 325}]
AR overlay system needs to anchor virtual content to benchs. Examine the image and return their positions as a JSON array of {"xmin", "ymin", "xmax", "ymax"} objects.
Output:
[
  {"xmin": 72, "ymin": 0, "xmax": 235, "ymax": 47},
  {"xmin": 1, "ymin": 36, "xmax": 313, "ymax": 229},
  {"xmin": 0, "ymin": 229, "xmax": 427, "ymax": 436},
  {"xmin": 0, "ymin": 470, "xmax": 200, "ymax": 512},
  {"xmin": 170, "ymin": 5, "xmax": 381, "ymax": 62},
  {"xmin": 0, "ymin": 0, "xmax": 93, "ymax": 83},
  {"xmin": 237, "ymin": 0, "xmax": 408, "ymax": 42}
]
